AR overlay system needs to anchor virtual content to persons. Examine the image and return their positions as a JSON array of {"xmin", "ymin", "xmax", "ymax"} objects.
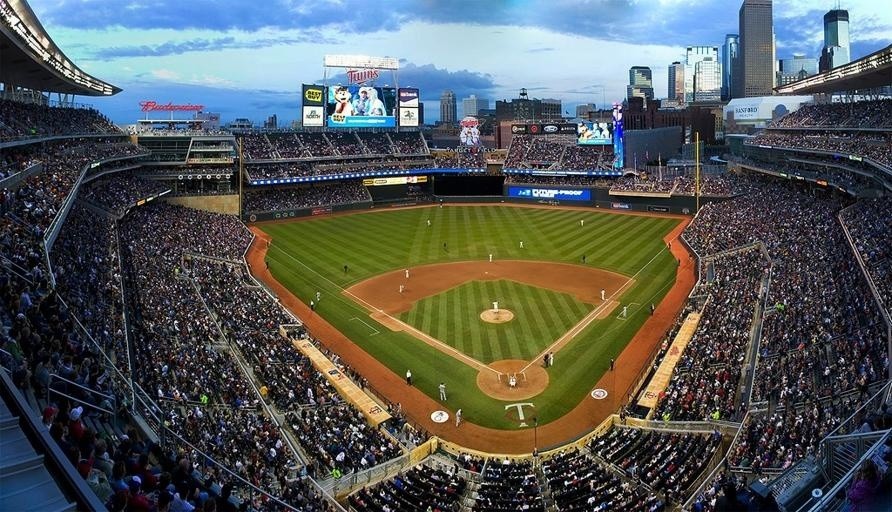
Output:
[
  {"xmin": 519, "ymin": 241, "xmax": 524, "ymax": 249},
  {"xmin": 600, "ymin": 288, "xmax": 605, "ymax": 300},
  {"xmin": 353, "ymin": 88, "xmax": 370, "ymax": 116},
  {"xmin": 492, "ymin": 299, "xmax": 500, "ymax": 316},
  {"xmin": 614, "ymin": 390, "xmax": 649, "ymax": 436},
  {"xmin": 455, "ymin": 408, "xmax": 463, "ymax": 427},
  {"xmin": 582, "ymin": 255, "xmax": 586, "ymax": 262},
  {"xmin": 310, "ymin": 299, "xmax": 314, "ymax": 311},
  {"xmin": 610, "ymin": 358, "xmax": 614, "ymax": 371},
  {"xmin": 316, "ymin": 289, "xmax": 321, "ymax": 302},
  {"xmin": 344, "ymin": 264, "xmax": 348, "ymax": 275},
  {"xmin": 509, "ymin": 376, "xmax": 517, "ymax": 388},
  {"xmin": 399, "ymin": 282, "xmax": 403, "ymax": 293},
  {"xmin": 362, "ymin": 90, "xmax": 386, "ymax": 116},
  {"xmin": 622, "ymin": 305, "xmax": 628, "ymax": 317},
  {"xmin": 489, "ymin": 254, "xmax": 493, "ymax": 262},
  {"xmin": 649, "ymin": 92, "xmax": 890, "ymax": 512},
  {"xmin": 288, "ymin": 318, "xmax": 426, "ymax": 512},
  {"xmin": 438, "ymin": 381, "xmax": 447, "ymax": 402},
  {"xmin": 580, "ymin": 219, "xmax": 584, "ymax": 226},
  {"xmin": 0, "ymin": 92, "xmax": 288, "ymax": 510},
  {"xmin": 544, "ymin": 353, "xmax": 549, "ymax": 366},
  {"xmin": 426, "ymin": 429, "xmax": 651, "ymax": 512},
  {"xmin": 428, "ymin": 219, "xmax": 431, "ymax": 228},
  {"xmin": 549, "ymin": 352, "xmax": 554, "ymax": 366},
  {"xmin": 288, "ymin": 125, "xmax": 650, "ymax": 208},
  {"xmin": 405, "ymin": 268, "xmax": 410, "ymax": 279}
]
